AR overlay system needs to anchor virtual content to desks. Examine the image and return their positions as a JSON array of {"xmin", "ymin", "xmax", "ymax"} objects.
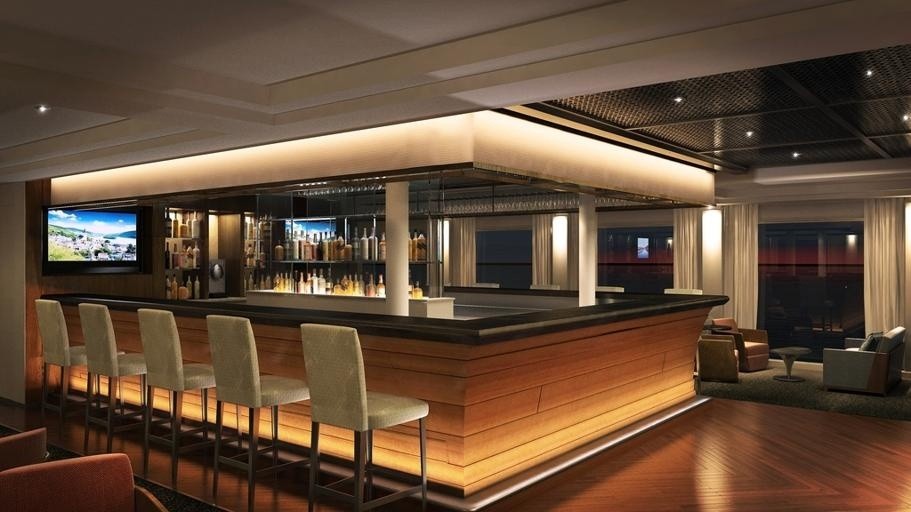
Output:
[
  {"xmin": 702, "ymin": 324, "xmax": 731, "ymax": 332},
  {"xmin": 770, "ymin": 347, "xmax": 812, "ymax": 382}
]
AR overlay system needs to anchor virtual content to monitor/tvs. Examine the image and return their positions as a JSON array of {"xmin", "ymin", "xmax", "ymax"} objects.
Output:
[{"xmin": 42, "ymin": 203, "xmax": 151, "ymax": 275}]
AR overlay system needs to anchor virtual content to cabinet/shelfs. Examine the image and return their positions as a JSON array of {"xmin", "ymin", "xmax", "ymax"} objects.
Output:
[
  {"xmin": 256, "ymin": 212, "xmax": 445, "ymax": 297},
  {"xmin": 219, "ymin": 213, "xmax": 270, "ymax": 297},
  {"xmin": 165, "ymin": 208, "xmax": 208, "ymax": 300}
]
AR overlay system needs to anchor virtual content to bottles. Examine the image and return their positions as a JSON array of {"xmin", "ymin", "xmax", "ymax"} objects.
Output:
[
  {"xmin": 165, "ymin": 241, "xmax": 201, "ymax": 271},
  {"xmin": 173, "ymin": 211, "xmax": 178, "ymax": 237},
  {"xmin": 180, "ymin": 211, "xmax": 188, "ymax": 237},
  {"xmin": 276, "ymin": 229, "xmax": 426, "ymax": 261},
  {"xmin": 245, "ymin": 212, "xmax": 271, "ymax": 289},
  {"xmin": 272, "ymin": 268, "xmax": 423, "ymax": 298},
  {"xmin": 187, "ymin": 212, "xmax": 192, "ymax": 238},
  {"xmin": 192, "ymin": 211, "xmax": 200, "ymax": 238},
  {"xmin": 165, "ymin": 273, "xmax": 203, "ymax": 300},
  {"xmin": 165, "ymin": 207, "xmax": 172, "ymax": 237}
]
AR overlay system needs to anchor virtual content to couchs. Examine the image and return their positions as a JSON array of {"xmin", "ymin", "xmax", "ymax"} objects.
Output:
[
  {"xmin": 700, "ymin": 334, "xmax": 739, "ymax": 382},
  {"xmin": 823, "ymin": 326, "xmax": 906, "ymax": 396},
  {"xmin": 712, "ymin": 318, "xmax": 769, "ymax": 372}
]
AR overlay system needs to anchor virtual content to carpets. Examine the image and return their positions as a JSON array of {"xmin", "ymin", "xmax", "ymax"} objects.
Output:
[
  {"xmin": 695, "ymin": 361, "xmax": 911, "ymax": 421},
  {"xmin": 0, "ymin": 422, "xmax": 231, "ymax": 512}
]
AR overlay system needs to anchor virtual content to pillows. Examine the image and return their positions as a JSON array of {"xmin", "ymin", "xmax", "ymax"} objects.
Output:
[{"xmin": 859, "ymin": 331, "xmax": 883, "ymax": 352}]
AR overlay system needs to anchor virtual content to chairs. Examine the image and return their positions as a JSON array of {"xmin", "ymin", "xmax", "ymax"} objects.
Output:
[
  {"xmin": 77, "ymin": 302, "xmax": 147, "ymax": 454},
  {"xmin": 1, "ymin": 453, "xmax": 170, "ymax": 511},
  {"xmin": 530, "ymin": 284, "xmax": 560, "ymax": 291},
  {"xmin": 137, "ymin": 309, "xmax": 242, "ymax": 491},
  {"xmin": 206, "ymin": 314, "xmax": 311, "ymax": 511},
  {"xmin": 0, "ymin": 428, "xmax": 50, "ymax": 473},
  {"xmin": 300, "ymin": 323, "xmax": 430, "ymax": 512},
  {"xmin": 664, "ymin": 288, "xmax": 702, "ymax": 376},
  {"xmin": 472, "ymin": 282, "xmax": 499, "ymax": 288},
  {"xmin": 34, "ymin": 299, "xmax": 125, "ymax": 440},
  {"xmin": 595, "ymin": 286, "xmax": 625, "ymax": 293}
]
